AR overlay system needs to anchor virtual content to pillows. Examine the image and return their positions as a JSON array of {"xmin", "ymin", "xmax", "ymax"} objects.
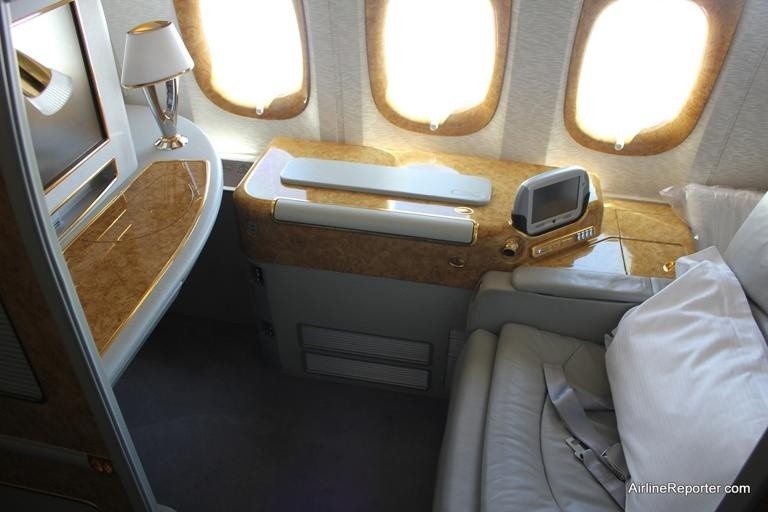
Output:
[{"xmin": 600, "ymin": 248, "xmax": 767, "ymax": 511}]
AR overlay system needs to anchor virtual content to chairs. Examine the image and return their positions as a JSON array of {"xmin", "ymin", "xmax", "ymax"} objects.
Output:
[{"xmin": 431, "ymin": 189, "xmax": 768, "ymax": 510}]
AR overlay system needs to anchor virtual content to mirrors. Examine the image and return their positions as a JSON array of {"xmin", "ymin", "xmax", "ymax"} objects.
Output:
[{"xmin": 11, "ymin": 3, "xmax": 110, "ymax": 198}]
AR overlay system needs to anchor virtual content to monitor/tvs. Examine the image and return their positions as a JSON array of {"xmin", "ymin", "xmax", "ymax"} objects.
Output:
[{"xmin": 1, "ymin": 1, "xmax": 138, "ymax": 251}]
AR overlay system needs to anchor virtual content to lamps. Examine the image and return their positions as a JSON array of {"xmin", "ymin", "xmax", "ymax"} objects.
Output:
[{"xmin": 123, "ymin": 21, "xmax": 193, "ymax": 151}]
